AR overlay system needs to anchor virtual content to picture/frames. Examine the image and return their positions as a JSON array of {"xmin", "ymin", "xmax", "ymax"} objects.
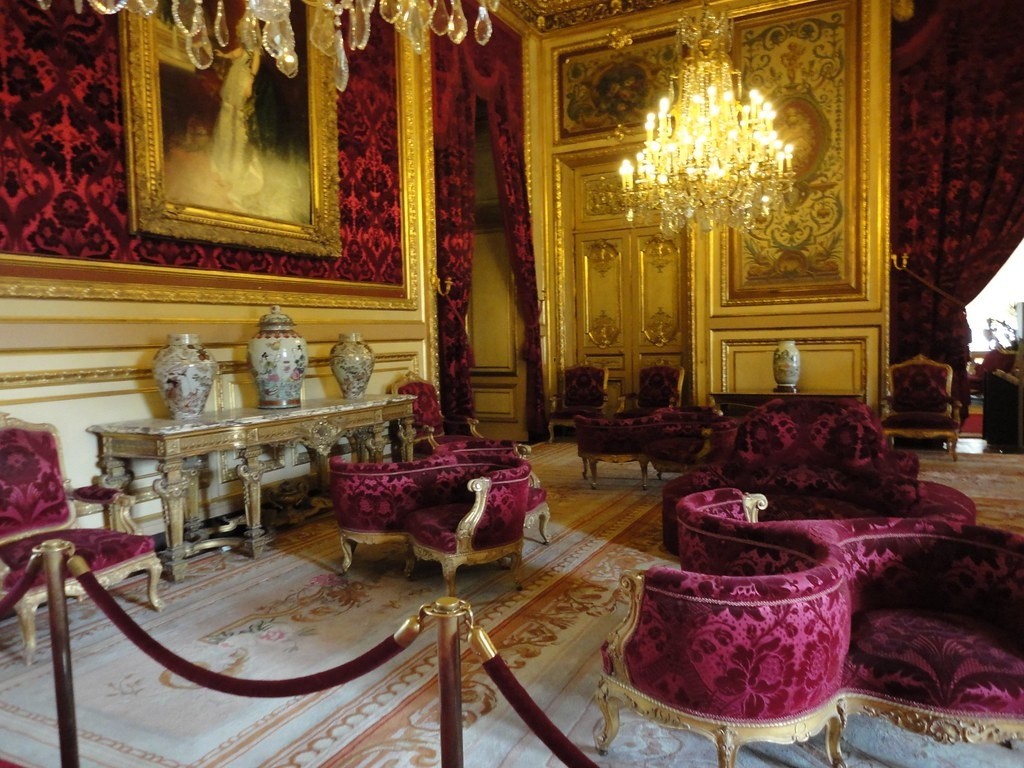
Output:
[{"xmin": 119, "ymin": 0, "xmax": 343, "ymax": 260}]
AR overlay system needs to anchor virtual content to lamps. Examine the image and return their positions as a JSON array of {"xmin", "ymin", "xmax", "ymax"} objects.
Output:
[{"xmin": 617, "ymin": 0, "xmax": 796, "ymax": 241}]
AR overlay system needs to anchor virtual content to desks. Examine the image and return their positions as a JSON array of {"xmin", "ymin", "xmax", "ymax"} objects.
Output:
[
  {"xmin": 708, "ymin": 387, "xmax": 865, "ymax": 418},
  {"xmin": 86, "ymin": 393, "xmax": 418, "ymax": 583}
]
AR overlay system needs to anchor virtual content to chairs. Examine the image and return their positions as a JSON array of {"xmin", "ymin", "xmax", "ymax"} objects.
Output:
[
  {"xmin": 0, "ymin": 412, "xmax": 167, "ymax": 669},
  {"xmin": 575, "ymin": 396, "xmax": 1024, "ymax": 768},
  {"xmin": 391, "ymin": 371, "xmax": 483, "ymax": 463},
  {"xmin": 615, "ymin": 358, "xmax": 685, "ymax": 414},
  {"xmin": 547, "ymin": 365, "xmax": 609, "ymax": 443},
  {"xmin": 877, "ymin": 353, "xmax": 962, "ymax": 464},
  {"xmin": 330, "ymin": 438, "xmax": 551, "ymax": 601}
]
